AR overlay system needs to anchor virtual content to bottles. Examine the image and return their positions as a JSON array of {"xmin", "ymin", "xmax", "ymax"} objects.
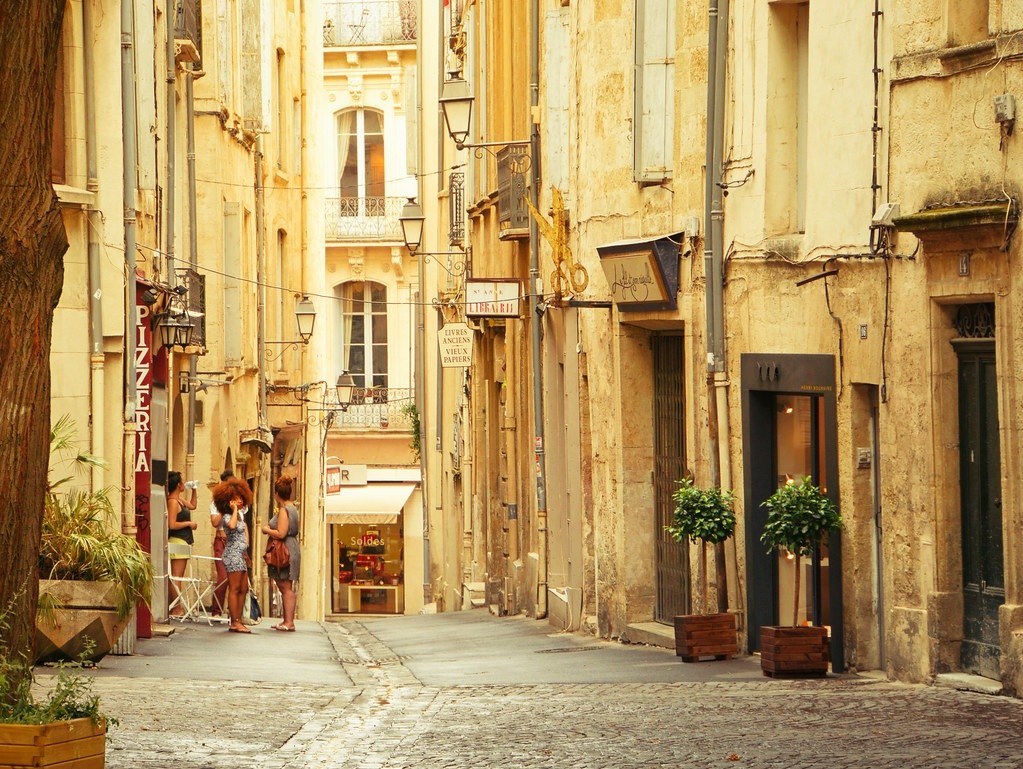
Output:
[
  {"xmin": 391, "ymin": 573, "xmax": 399, "ymax": 586},
  {"xmin": 184, "ymin": 480, "xmax": 201, "ymax": 490}
]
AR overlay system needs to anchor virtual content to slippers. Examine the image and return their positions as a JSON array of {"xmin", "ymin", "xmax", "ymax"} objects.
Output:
[
  {"xmin": 276, "ymin": 624, "xmax": 295, "ymax": 631},
  {"xmin": 271, "ymin": 622, "xmax": 285, "ymax": 628}
]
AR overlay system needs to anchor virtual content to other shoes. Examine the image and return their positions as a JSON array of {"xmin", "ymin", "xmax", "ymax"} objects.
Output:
[
  {"xmin": 229, "ymin": 624, "xmax": 251, "ymax": 633},
  {"xmin": 169, "ymin": 607, "xmax": 192, "ymax": 620},
  {"xmin": 210, "ymin": 614, "xmax": 223, "ymax": 624}
]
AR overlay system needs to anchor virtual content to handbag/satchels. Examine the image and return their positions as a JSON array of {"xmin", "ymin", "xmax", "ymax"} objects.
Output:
[
  {"xmin": 263, "ymin": 506, "xmax": 290, "ymax": 568},
  {"xmin": 241, "ymin": 577, "xmax": 262, "ymax": 625}
]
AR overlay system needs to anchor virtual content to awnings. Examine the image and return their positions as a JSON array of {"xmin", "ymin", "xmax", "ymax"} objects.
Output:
[{"xmin": 324, "ymin": 482, "xmax": 417, "ymax": 524}]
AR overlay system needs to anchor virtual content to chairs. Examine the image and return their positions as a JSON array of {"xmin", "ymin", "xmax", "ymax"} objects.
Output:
[{"xmin": 167, "ymin": 542, "xmax": 226, "ymax": 627}]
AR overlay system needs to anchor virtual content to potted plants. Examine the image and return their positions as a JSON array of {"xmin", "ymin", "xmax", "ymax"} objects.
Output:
[
  {"xmin": 0, "ymin": 566, "xmax": 120, "ymax": 769},
  {"xmin": 760, "ymin": 475, "xmax": 843, "ymax": 678},
  {"xmin": 35, "ymin": 412, "xmax": 155, "ymax": 668},
  {"xmin": 664, "ymin": 477, "xmax": 739, "ymax": 661}
]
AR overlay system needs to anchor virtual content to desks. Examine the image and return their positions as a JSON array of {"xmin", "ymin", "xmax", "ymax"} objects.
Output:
[{"xmin": 347, "ymin": 585, "xmax": 404, "ymax": 614}]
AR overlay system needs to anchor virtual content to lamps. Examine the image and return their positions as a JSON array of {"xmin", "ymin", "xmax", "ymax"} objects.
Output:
[
  {"xmin": 150, "ymin": 310, "xmax": 180, "ymax": 357},
  {"xmin": 305, "ymin": 369, "xmax": 356, "ymax": 432},
  {"xmin": 263, "ymin": 293, "xmax": 316, "ymax": 361},
  {"xmin": 439, "ymin": 69, "xmax": 533, "ymax": 174},
  {"xmin": 172, "ymin": 309, "xmax": 196, "ymax": 352},
  {"xmin": 399, "ymin": 198, "xmax": 473, "ymax": 283},
  {"xmin": 142, "ymin": 288, "xmax": 163, "ymax": 306},
  {"xmin": 170, "ymin": 284, "xmax": 188, "ymax": 297}
]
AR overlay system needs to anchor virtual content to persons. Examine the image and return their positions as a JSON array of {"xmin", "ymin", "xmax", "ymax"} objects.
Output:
[
  {"xmin": 167, "ymin": 470, "xmax": 198, "ymax": 616},
  {"xmin": 209, "ymin": 470, "xmax": 252, "ymax": 633},
  {"xmin": 261, "ymin": 475, "xmax": 302, "ymax": 631}
]
